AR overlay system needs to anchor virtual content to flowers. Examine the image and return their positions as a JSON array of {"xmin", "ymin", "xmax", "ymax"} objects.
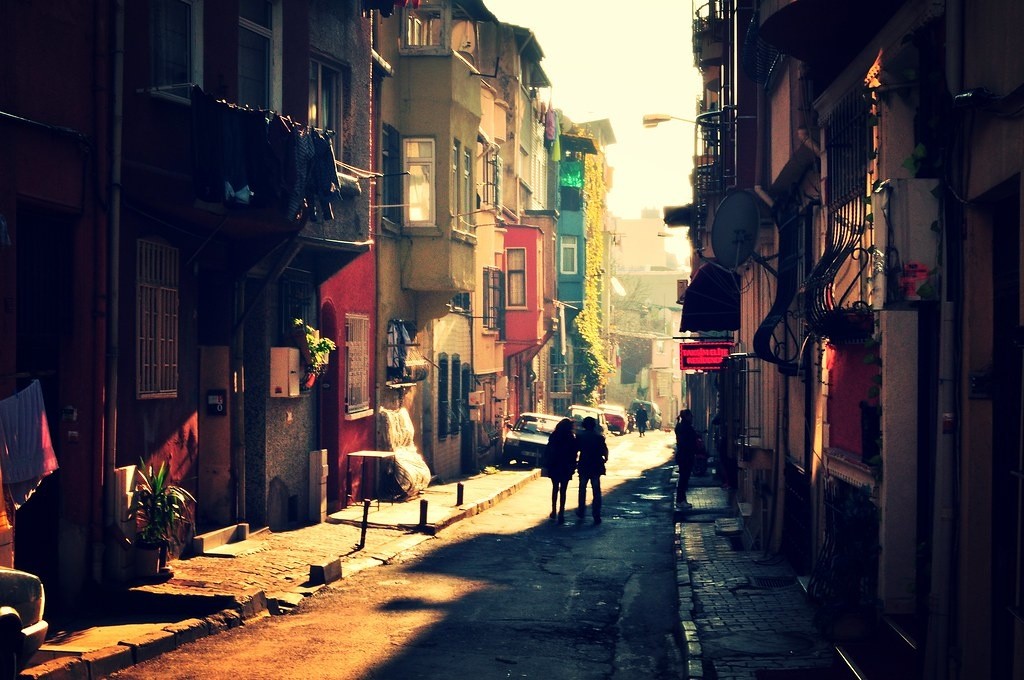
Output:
[{"xmin": 292, "ymin": 319, "xmax": 338, "ymax": 370}]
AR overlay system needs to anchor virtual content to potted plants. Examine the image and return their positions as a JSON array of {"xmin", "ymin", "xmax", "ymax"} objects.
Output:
[{"xmin": 120, "ymin": 456, "xmax": 198, "ymax": 581}]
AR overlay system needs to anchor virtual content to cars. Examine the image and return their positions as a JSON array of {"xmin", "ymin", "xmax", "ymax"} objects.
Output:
[
  {"xmin": 502, "ymin": 412, "xmax": 566, "ymax": 464},
  {"xmin": 565, "ymin": 405, "xmax": 608, "ymax": 437},
  {"xmin": 0, "ymin": 565, "xmax": 49, "ymax": 680},
  {"xmin": 598, "ymin": 404, "xmax": 627, "ymax": 436}
]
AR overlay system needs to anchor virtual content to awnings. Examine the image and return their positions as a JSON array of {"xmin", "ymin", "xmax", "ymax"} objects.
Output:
[{"xmin": 678, "ymin": 261, "xmax": 740, "ymax": 333}]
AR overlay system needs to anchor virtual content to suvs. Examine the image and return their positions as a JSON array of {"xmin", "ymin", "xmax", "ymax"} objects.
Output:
[{"xmin": 629, "ymin": 399, "xmax": 663, "ymax": 431}]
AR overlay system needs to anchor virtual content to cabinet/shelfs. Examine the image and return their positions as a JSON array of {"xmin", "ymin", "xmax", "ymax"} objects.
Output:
[{"xmin": 270, "ymin": 348, "xmax": 300, "ymax": 397}]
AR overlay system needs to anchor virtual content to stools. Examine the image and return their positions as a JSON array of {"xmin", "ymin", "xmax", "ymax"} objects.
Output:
[{"xmin": 345, "ymin": 451, "xmax": 395, "ymax": 511}]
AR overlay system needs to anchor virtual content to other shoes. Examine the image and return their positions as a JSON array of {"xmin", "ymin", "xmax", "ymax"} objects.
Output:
[
  {"xmin": 550, "ymin": 512, "xmax": 556, "ymax": 519},
  {"xmin": 594, "ymin": 518, "xmax": 601, "ymax": 524},
  {"xmin": 576, "ymin": 510, "xmax": 584, "ymax": 518},
  {"xmin": 558, "ymin": 515, "xmax": 564, "ymax": 523},
  {"xmin": 676, "ymin": 501, "xmax": 692, "ymax": 508}
]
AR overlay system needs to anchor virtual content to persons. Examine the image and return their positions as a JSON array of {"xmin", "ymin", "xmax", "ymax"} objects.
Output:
[
  {"xmin": 636, "ymin": 404, "xmax": 648, "ymax": 437},
  {"xmin": 675, "ymin": 409, "xmax": 701, "ymax": 509},
  {"xmin": 541, "ymin": 419, "xmax": 578, "ymax": 523},
  {"xmin": 574, "ymin": 417, "xmax": 608, "ymax": 525}
]
